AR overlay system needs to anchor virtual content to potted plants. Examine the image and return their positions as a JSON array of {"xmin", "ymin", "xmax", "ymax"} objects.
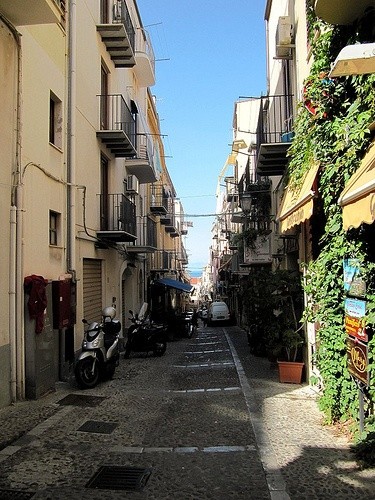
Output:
[{"xmin": 267, "ymin": 267, "xmax": 307, "ymax": 385}]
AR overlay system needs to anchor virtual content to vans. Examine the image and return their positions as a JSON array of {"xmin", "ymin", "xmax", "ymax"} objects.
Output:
[{"xmin": 207, "ymin": 302, "xmax": 231, "ymax": 326}]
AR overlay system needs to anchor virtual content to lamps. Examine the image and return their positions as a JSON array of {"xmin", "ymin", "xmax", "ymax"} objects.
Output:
[{"xmin": 327, "ymin": 42, "xmax": 375, "ymax": 77}]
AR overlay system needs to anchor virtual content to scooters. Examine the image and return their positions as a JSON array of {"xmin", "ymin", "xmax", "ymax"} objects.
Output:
[
  {"xmin": 73, "ymin": 304, "xmax": 128, "ymax": 389},
  {"xmin": 123, "ymin": 309, "xmax": 168, "ymax": 359}
]
